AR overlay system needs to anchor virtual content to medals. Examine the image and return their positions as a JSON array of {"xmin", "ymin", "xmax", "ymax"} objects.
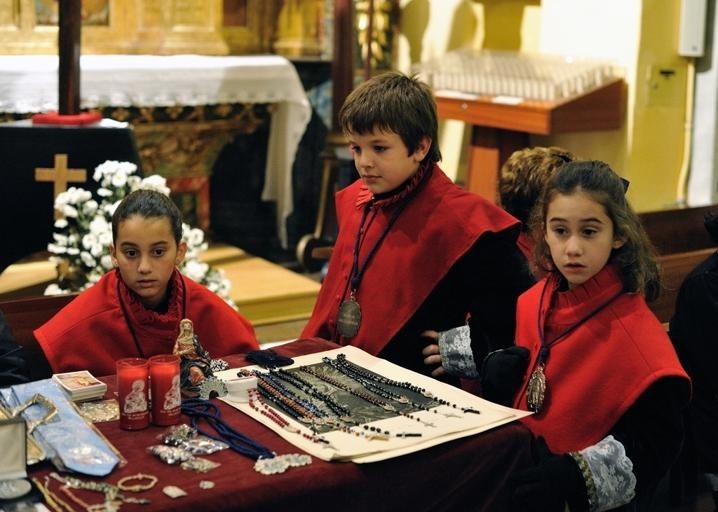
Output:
[
  {"xmin": 525, "ymin": 367, "xmax": 547, "ymax": 414},
  {"xmin": 334, "ymin": 296, "xmax": 361, "ymax": 340}
]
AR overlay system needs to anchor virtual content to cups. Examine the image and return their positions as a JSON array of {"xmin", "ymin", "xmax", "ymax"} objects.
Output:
[
  {"xmin": 147, "ymin": 356, "xmax": 180, "ymax": 426},
  {"xmin": 115, "ymin": 359, "xmax": 148, "ymax": 430}
]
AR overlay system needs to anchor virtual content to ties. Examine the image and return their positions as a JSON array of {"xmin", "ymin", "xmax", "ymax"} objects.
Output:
[
  {"xmin": 11, "ymin": 378, "xmax": 120, "ymax": 477},
  {"xmin": 0, "ymin": 386, "xmax": 73, "ymax": 479}
]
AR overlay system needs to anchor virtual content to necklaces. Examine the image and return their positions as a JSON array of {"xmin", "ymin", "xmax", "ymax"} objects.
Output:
[
  {"xmin": 182, "ymin": 353, "xmax": 480, "ymax": 474},
  {"xmin": 116, "ymin": 269, "xmax": 187, "ymax": 361},
  {"xmin": 30, "ymin": 469, "xmax": 125, "ymax": 512}
]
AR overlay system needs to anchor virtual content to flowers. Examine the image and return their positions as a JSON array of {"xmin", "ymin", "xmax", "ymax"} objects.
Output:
[{"xmin": 41, "ymin": 159, "xmax": 242, "ymax": 316}]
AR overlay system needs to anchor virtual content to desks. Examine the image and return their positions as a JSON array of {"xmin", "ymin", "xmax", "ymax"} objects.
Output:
[{"xmin": 1, "ymin": 337, "xmax": 535, "ymax": 512}]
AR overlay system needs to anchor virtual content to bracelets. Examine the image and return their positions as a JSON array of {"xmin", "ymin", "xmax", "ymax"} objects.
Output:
[{"xmin": 119, "ymin": 472, "xmax": 158, "ymax": 493}]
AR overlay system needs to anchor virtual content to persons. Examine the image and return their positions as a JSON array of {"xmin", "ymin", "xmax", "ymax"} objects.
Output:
[
  {"xmin": 670, "ymin": 249, "xmax": 715, "ymax": 473},
  {"xmin": 498, "ymin": 144, "xmax": 584, "ymax": 284},
  {"xmin": 481, "ymin": 159, "xmax": 693, "ymax": 512},
  {"xmin": 2, "ymin": 187, "xmax": 260, "ymax": 390},
  {"xmin": 297, "ymin": 71, "xmax": 538, "ymax": 393}
]
